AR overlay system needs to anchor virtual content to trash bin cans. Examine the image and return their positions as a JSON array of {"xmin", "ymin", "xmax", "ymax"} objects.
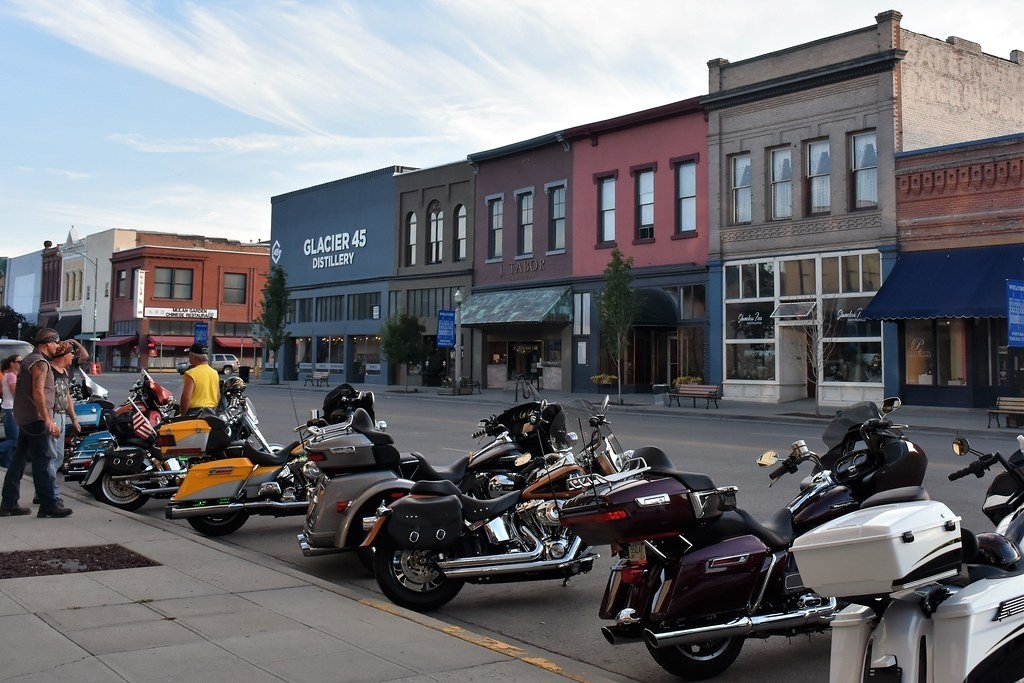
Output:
[{"xmin": 238, "ymin": 366, "xmax": 250, "ymax": 383}]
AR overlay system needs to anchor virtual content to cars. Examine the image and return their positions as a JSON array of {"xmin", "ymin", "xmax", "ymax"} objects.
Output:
[{"xmin": 0, "ymin": 339, "xmax": 34, "ymax": 367}]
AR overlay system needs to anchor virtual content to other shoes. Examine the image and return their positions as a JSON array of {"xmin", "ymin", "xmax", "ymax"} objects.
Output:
[
  {"xmin": 57, "ymin": 497, "xmax": 64, "ymax": 507},
  {"xmin": 33, "ymin": 498, "xmax": 40, "ymax": 503}
]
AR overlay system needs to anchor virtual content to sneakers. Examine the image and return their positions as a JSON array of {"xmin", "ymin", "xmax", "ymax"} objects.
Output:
[
  {"xmin": 37, "ymin": 505, "xmax": 73, "ymax": 518},
  {"xmin": 0, "ymin": 503, "xmax": 31, "ymax": 516}
]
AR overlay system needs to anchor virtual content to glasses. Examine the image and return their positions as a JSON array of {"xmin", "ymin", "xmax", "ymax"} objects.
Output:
[
  {"xmin": 71, "ymin": 351, "xmax": 75, "ymax": 354},
  {"xmin": 15, "ymin": 360, "xmax": 22, "ymax": 364},
  {"xmin": 54, "ymin": 338, "xmax": 60, "ymax": 344}
]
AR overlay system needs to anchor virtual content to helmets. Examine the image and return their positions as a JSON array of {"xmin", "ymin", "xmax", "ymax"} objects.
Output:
[
  {"xmin": 976, "ymin": 532, "xmax": 1021, "ymax": 570},
  {"xmin": 223, "ymin": 376, "xmax": 246, "ymax": 392}
]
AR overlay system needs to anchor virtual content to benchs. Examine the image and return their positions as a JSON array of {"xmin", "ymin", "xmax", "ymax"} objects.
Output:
[
  {"xmin": 304, "ymin": 372, "xmax": 330, "ymax": 387},
  {"xmin": 668, "ymin": 382, "xmax": 722, "ymax": 410},
  {"xmin": 986, "ymin": 396, "xmax": 1024, "ymax": 428}
]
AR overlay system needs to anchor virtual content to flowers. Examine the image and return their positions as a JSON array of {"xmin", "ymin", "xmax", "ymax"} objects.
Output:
[
  {"xmin": 590, "ymin": 374, "xmax": 618, "ymax": 383},
  {"xmin": 672, "ymin": 376, "xmax": 703, "ymax": 388},
  {"xmin": 441, "ymin": 376, "xmax": 468, "ymax": 388}
]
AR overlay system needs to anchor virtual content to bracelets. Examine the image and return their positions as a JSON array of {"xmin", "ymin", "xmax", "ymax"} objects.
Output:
[{"xmin": 71, "ymin": 416, "xmax": 77, "ymax": 420}]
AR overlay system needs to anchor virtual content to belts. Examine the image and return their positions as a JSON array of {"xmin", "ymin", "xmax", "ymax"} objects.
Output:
[
  {"xmin": 189, "ymin": 407, "xmax": 201, "ymax": 411},
  {"xmin": 54, "ymin": 411, "xmax": 66, "ymax": 414}
]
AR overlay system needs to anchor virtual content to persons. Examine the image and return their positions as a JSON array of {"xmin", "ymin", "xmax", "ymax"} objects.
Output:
[
  {"xmin": 179, "ymin": 345, "xmax": 221, "ymax": 417},
  {"xmin": 0, "ymin": 354, "xmax": 22, "ymax": 468},
  {"xmin": 32, "ymin": 339, "xmax": 89, "ymax": 504},
  {"xmin": 0, "ymin": 328, "xmax": 74, "ymax": 518}
]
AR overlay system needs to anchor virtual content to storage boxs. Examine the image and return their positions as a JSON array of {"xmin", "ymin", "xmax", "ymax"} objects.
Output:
[
  {"xmin": 788, "ymin": 500, "xmax": 963, "ymax": 599},
  {"xmin": 155, "ymin": 420, "xmax": 212, "ymax": 455},
  {"xmin": 304, "ymin": 434, "xmax": 376, "ymax": 475},
  {"xmin": 65, "ymin": 404, "xmax": 102, "ymax": 426},
  {"xmin": 559, "ymin": 477, "xmax": 695, "ymax": 545}
]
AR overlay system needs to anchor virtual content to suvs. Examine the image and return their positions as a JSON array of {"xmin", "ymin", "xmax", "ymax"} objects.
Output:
[{"xmin": 177, "ymin": 354, "xmax": 240, "ymax": 375}]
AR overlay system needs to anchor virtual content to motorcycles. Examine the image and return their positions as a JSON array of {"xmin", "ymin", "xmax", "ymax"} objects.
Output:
[
  {"xmin": 62, "ymin": 365, "xmax": 284, "ymax": 510},
  {"xmin": 790, "ymin": 436, "xmax": 1024, "ymax": 683},
  {"xmin": 153, "ymin": 382, "xmax": 387, "ymax": 537},
  {"xmin": 296, "ymin": 377, "xmax": 576, "ymax": 581},
  {"xmin": 562, "ymin": 398, "xmax": 927, "ymax": 683},
  {"xmin": 362, "ymin": 380, "xmax": 671, "ymax": 610}
]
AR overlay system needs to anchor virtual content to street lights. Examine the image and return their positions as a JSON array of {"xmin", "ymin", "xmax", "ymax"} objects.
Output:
[
  {"xmin": 455, "ymin": 287, "xmax": 462, "ymax": 392},
  {"xmin": 56, "ymin": 251, "xmax": 98, "ymax": 363},
  {"xmin": 18, "ymin": 323, "xmax": 23, "ymax": 340}
]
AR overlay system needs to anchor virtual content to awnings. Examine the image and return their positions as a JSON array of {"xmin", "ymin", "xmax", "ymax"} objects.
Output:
[
  {"xmin": 148, "ymin": 336, "xmax": 194, "ymax": 346},
  {"xmin": 454, "ymin": 285, "xmax": 574, "ymax": 325},
  {"xmin": 74, "ymin": 333, "xmax": 102, "ymax": 340},
  {"xmin": 96, "ymin": 336, "xmax": 136, "ymax": 346},
  {"xmin": 862, "ymin": 243, "xmax": 1024, "ymax": 321},
  {"xmin": 214, "ymin": 336, "xmax": 263, "ymax": 347},
  {"xmin": 52, "ymin": 315, "xmax": 82, "ymax": 341}
]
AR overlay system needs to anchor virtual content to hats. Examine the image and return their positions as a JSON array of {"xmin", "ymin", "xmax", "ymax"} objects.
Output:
[{"xmin": 184, "ymin": 343, "xmax": 206, "ymax": 354}]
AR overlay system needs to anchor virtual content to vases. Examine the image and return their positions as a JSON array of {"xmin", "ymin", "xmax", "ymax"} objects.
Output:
[
  {"xmin": 437, "ymin": 386, "xmax": 473, "ymax": 395},
  {"xmin": 683, "ymin": 381, "xmax": 698, "ymax": 385},
  {"xmin": 600, "ymin": 379, "xmax": 612, "ymax": 384}
]
